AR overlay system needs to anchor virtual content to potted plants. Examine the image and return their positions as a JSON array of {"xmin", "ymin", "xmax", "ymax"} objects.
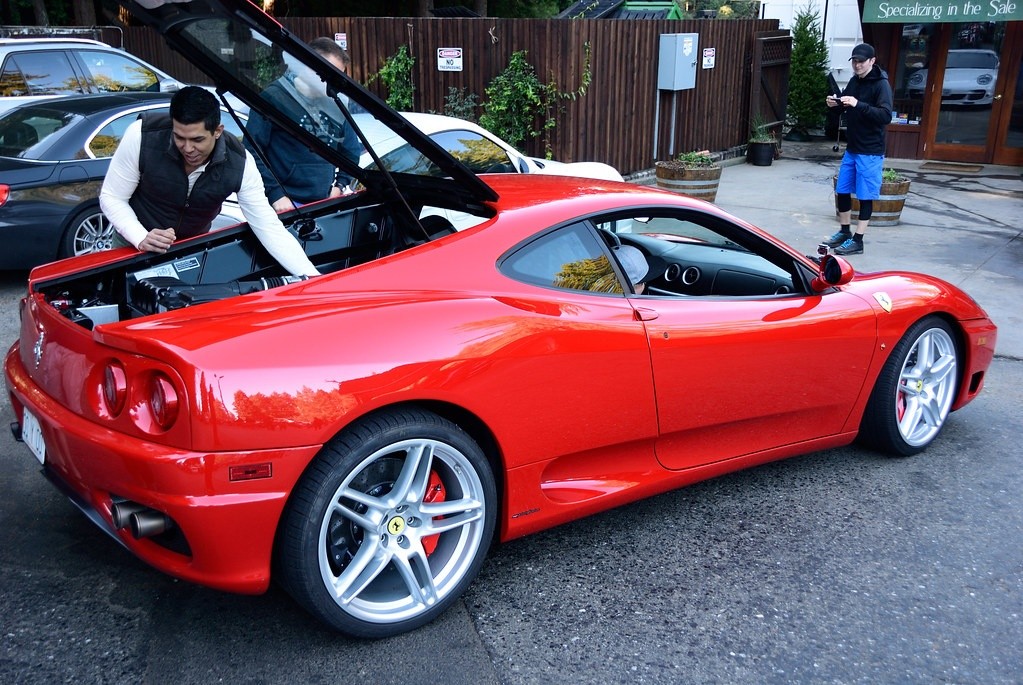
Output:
[
  {"xmin": 743, "ymin": 110, "xmax": 776, "ymax": 167},
  {"xmin": 652, "ymin": 150, "xmax": 721, "ymax": 204},
  {"xmin": 832, "ymin": 167, "xmax": 911, "ymax": 228}
]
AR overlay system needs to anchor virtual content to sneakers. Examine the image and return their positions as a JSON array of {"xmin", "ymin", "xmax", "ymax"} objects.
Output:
[
  {"xmin": 834, "ymin": 238, "xmax": 864, "ymax": 254},
  {"xmin": 822, "ymin": 231, "xmax": 852, "ymax": 247}
]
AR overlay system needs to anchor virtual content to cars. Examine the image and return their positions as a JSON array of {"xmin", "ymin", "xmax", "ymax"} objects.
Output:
[
  {"xmin": 0, "ymin": 91, "xmax": 260, "ymax": 277},
  {"xmin": 207, "ymin": 110, "xmax": 628, "ymax": 251},
  {"xmin": 906, "ymin": 49, "xmax": 1000, "ymax": 110}
]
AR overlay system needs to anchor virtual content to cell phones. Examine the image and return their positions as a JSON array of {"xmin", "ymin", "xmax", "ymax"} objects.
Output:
[{"xmin": 831, "ymin": 98, "xmax": 845, "ymax": 105}]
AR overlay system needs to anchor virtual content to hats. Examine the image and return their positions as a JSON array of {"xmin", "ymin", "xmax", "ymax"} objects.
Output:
[{"xmin": 848, "ymin": 43, "xmax": 875, "ymax": 61}]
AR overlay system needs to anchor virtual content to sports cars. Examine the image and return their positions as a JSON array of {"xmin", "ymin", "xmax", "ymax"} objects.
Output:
[{"xmin": 4, "ymin": 1, "xmax": 998, "ymax": 644}]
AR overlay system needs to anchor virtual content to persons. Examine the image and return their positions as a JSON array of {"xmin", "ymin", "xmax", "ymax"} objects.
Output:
[
  {"xmin": 822, "ymin": 44, "xmax": 892, "ymax": 255},
  {"xmin": 241, "ymin": 37, "xmax": 361, "ymax": 214},
  {"xmin": 611, "ymin": 245, "xmax": 667, "ymax": 295},
  {"xmin": 99, "ymin": 85, "xmax": 322, "ymax": 281}
]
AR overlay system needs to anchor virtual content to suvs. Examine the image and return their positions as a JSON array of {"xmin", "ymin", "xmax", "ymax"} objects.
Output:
[{"xmin": 0, "ymin": 38, "xmax": 258, "ymax": 128}]
